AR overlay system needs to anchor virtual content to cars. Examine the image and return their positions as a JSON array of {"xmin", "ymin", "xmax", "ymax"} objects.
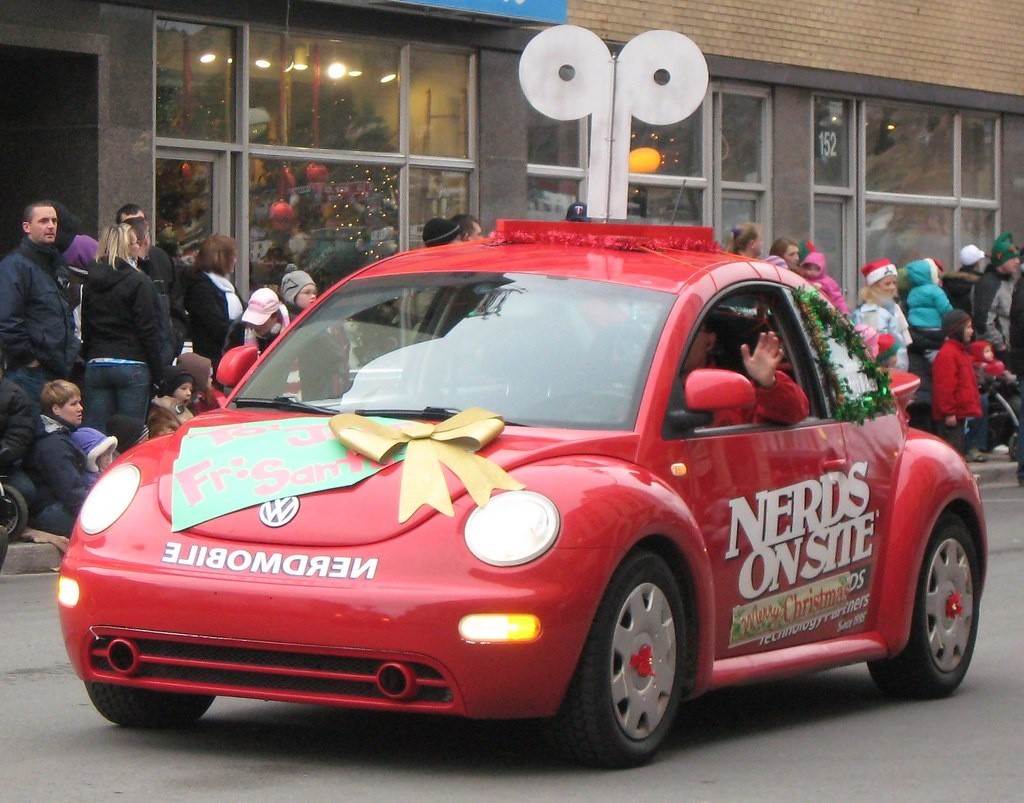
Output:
[{"xmin": 55, "ymin": 216, "xmax": 990, "ymax": 773}]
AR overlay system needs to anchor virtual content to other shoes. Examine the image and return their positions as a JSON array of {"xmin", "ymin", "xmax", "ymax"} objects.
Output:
[{"xmin": 966, "ymin": 448, "xmax": 986, "ymax": 462}]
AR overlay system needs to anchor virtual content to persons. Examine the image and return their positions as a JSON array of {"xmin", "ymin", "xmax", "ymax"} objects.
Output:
[
  {"xmin": 422, "ymin": 213, "xmax": 485, "ymax": 247},
  {"xmin": 0, "ymin": 199, "xmax": 350, "ymax": 572},
  {"xmin": 848, "ymin": 232, "xmax": 1024, "ymax": 489},
  {"xmin": 566, "ymin": 201, "xmax": 592, "ymax": 222},
  {"xmin": 677, "ymin": 307, "xmax": 811, "ymax": 429},
  {"xmin": 726, "ymin": 220, "xmax": 850, "ymax": 320}
]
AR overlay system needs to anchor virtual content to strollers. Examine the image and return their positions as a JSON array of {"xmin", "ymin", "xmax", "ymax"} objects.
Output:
[{"xmin": 968, "ymin": 364, "xmax": 1022, "ymax": 463}]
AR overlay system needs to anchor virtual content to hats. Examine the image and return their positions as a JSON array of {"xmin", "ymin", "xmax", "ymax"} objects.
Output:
[
  {"xmin": 566, "ymin": 201, "xmax": 590, "ymax": 222},
  {"xmin": 422, "ymin": 217, "xmax": 461, "ymax": 246},
  {"xmin": 765, "ymin": 255, "xmax": 788, "ymax": 269},
  {"xmin": 157, "ymin": 365, "xmax": 195, "ymax": 398},
  {"xmin": 241, "ymin": 288, "xmax": 279, "ymax": 325},
  {"xmin": 877, "ymin": 334, "xmax": 901, "ymax": 360},
  {"xmin": 959, "ymin": 244, "xmax": 985, "ymax": 266},
  {"xmin": 990, "ymin": 231, "xmax": 1019, "ymax": 267},
  {"xmin": 281, "ymin": 264, "xmax": 316, "ymax": 305},
  {"xmin": 854, "ymin": 324, "xmax": 879, "ymax": 359},
  {"xmin": 861, "ymin": 257, "xmax": 898, "ymax": 287},
  {"xmin": 970, "ymin": 340, "xmax": 995, "ymax": 363},
  {"xmin": 941, "ymin": 309, "xmax": 972, "ymax": 336}
]
